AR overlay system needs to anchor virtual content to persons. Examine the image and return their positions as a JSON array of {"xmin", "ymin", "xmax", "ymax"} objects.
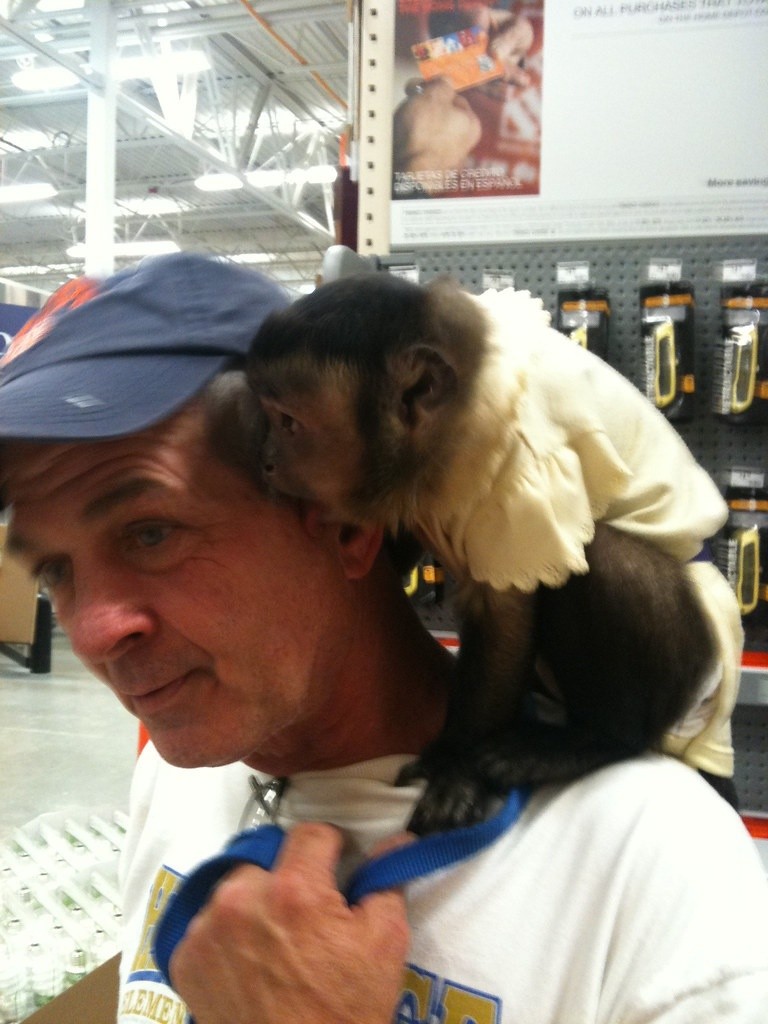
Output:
[
  {"xmin": 394, "ymin": 0, "xmax": 543, "ymax": 199},
  {"xmin": 0, "ymin": 255, "xmax": 768, "ymax": 1024}
]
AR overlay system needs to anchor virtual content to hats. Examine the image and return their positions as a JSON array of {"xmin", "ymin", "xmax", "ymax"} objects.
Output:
[{"xmin": 1, "ymin": 252, "xmax": 293, "ymax": 439}]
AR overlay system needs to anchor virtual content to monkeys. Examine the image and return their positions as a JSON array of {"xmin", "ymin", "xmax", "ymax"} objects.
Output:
[{"xmin": 245, "ymin": 270, "xmax": 729, "ymax": 834}]
